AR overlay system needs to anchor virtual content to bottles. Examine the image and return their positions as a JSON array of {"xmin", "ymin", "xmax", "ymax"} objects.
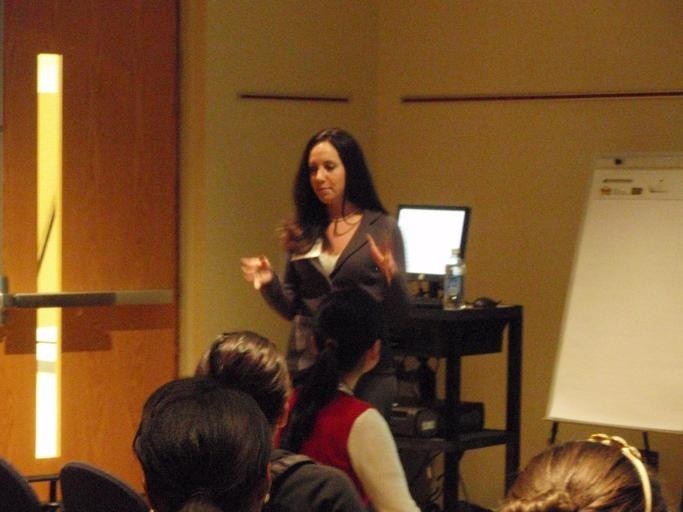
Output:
[{"xmin": 443, "ymin": 249, "xmax": 466, "ymax": 310}]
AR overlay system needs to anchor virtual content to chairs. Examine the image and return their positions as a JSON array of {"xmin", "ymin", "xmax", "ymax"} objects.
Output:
[
  {"xmin": 58, "ymin": 463, "xmax": 148, "ymax": 512},
  {"xmin": 0, "ymin": 457, "xmax": 61, "ymax": 512}
]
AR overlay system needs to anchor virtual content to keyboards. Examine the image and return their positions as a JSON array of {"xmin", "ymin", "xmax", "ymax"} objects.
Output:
[{"xmin": 405, "ymin": 292, "xmax": 445, "ymax": 307}]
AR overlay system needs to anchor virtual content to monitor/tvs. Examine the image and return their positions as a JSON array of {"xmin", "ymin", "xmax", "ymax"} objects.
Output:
[{"xmin": 395, "ymin": 204, "xmax": 470, "ymax": 295}]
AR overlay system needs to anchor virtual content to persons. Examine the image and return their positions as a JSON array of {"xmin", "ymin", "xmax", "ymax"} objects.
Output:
[
  {"xmin": 268, "ymin": 288, "xmax": 426, "ymax": 512},
  {"xmin": 191, "ymin": 329, "xmax": 365, "ymax": 512},
  {"xmin": 497, "ymin": 429, "xmax": 676, "ymax": 512},
  {"xmin": 131, "ymin": 374, "xmax": 278, "ymax": 510},
  {"xmin": 238, "ymin": 125, "xmax": 410, "ymax": 427}
]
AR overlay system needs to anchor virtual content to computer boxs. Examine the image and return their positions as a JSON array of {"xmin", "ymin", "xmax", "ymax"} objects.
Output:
[{"xmin": 387, "ymin": 394, "xmax": 484, "ymax": 440}]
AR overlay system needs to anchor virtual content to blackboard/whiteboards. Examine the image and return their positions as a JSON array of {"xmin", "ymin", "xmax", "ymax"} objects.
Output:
[{"xmin": 542, "ymin": 154, "xmax": 683, "ymax": 435}]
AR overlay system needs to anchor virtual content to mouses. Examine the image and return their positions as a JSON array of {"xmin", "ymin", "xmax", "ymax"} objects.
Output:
[{"xmin": 475, "ymin": 296, "xmax": 496, "ymax": 309}]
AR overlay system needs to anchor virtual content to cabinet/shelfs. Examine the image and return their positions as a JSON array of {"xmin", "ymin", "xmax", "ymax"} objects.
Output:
[{"xmin": 397, "ymin": 304, "xmax": 523, "ymax": 512}]
